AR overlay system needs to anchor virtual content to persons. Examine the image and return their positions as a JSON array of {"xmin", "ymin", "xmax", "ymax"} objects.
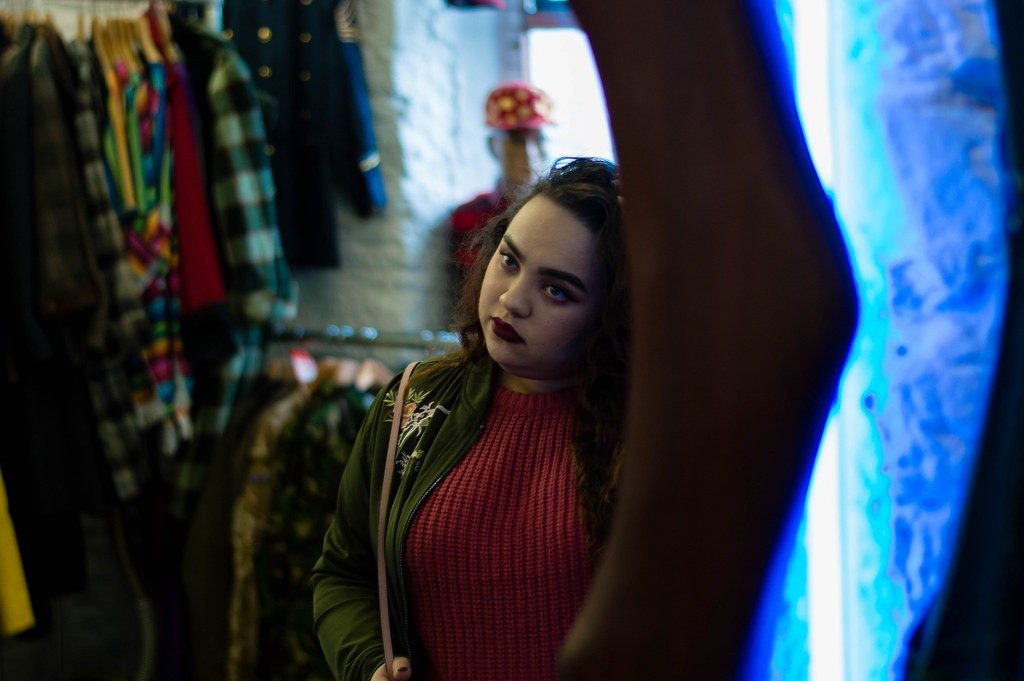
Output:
[
  {"xmin": 448, "ymin": 82, "xmax": 554, "ymax": 328},
  {"xmin": 310, "ymin": 150, "xmax": 630, "ymax": 681}
]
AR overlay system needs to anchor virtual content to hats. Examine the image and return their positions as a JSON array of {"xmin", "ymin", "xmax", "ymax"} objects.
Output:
[{"xmin": 485, "ymin": 84, "xmax": 551, "ymax": 130}]
